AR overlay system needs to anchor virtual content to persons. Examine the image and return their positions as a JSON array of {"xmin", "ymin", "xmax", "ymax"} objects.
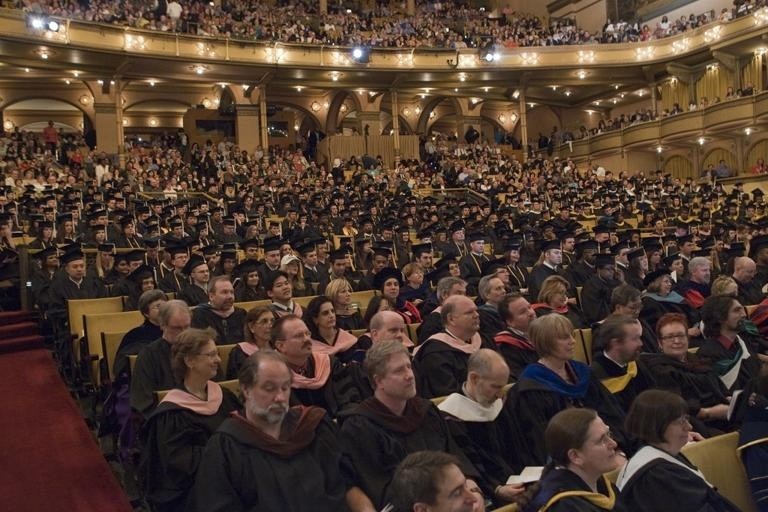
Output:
[
  {"xmin": 0, "ymin": 1, "xmax": 768, "ymax": 47},
  {"xmin": 0, "ymin": 85, "xmax": 767, "ymax": 512}
]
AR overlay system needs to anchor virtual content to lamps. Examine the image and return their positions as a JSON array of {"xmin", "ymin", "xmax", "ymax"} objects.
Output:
[
  {"xmin": 26, "ymin": 12, "xmax": 62, "ymax": 33},
  {"xmin": 352, "ymin": 45, "xmax": 372, "ymax": 64},
  {"xmin": 478, "ymin": 49, "xmax": 497, "ymax": 63}
]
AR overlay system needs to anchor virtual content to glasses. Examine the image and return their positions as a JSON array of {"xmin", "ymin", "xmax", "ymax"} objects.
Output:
[{"xmin": 660, "ymin": 334, "xmax": 686, "ymax": 340}]
